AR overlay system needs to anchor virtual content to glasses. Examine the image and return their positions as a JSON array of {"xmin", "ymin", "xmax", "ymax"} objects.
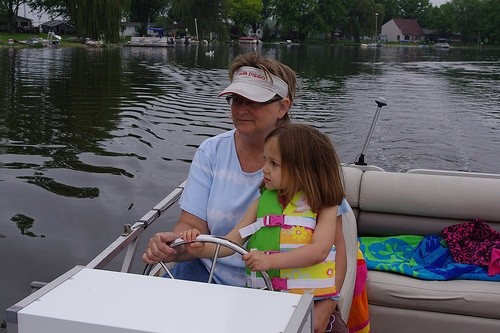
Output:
[{"xmin": 225, "ymin": 92, "xmax": 283, "ymax": 106}]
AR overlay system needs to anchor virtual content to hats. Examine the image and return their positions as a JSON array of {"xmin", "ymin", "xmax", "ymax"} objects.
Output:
[{"xmin": 218, "ymin": 66, "xmax": 289, "ymax": 103}]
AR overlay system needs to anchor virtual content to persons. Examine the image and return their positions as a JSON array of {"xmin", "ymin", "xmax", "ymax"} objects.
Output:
[
  {"xmin": 142, "ymin": 52, "xmax": 350, "ymax": 333},
  {"xmin": 180, "ymin": 124, "xmax": 345, "ymax": 296}
]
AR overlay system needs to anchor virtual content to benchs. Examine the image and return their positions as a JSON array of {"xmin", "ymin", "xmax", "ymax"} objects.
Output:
[{"xmin": 339, "ymin": 166, "xmax": 500, "ymax": 333}]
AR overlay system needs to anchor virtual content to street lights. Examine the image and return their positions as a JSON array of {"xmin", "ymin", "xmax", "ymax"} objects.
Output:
[{"xmin": 375, "ymin": 12, "xmax": 379, "ymax": 43}]
